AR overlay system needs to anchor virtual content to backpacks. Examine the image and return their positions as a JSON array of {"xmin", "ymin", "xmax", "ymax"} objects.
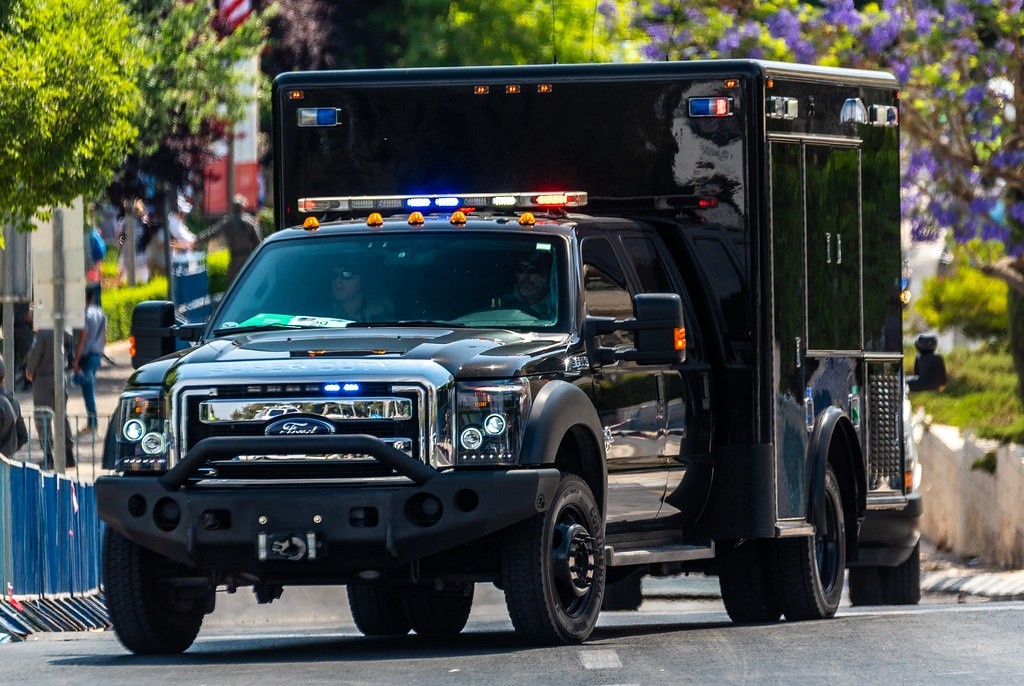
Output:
[{"xmin": 135, "ymin": 216, "xmax": 153, "ymax": 254}]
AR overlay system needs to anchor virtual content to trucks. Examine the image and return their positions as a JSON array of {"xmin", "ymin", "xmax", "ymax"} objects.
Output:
[
  {"xmin": 595, "ymin": 336, "xmax": 948, "ymax": 611},
  {"xmin": 94, "ymin": 56, "xmax": 924, "ymax": 659}
]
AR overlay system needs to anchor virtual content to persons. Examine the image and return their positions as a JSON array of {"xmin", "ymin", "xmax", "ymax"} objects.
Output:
[
  {"xmin": 147, "ymin": 196, "xmax": 197, "ymax": 281},
  {"xmin": 516, "ymin": 255, "xmax": 550, "ymax": 314},
  {"xmin": 74, "ymin": 288, "xmax": 108, "ymax": 434},
  {"xmin": 25, "ymin": 327, "xmax": 77, "ymax": 469},
  {"xmin": 187, "ymin": 194, "xmax": 261, "ymax": 277},
  {"xmin": 330, "ymin": 257, "xmax": 387, "ymax": 323},
  {"xmin": 0, "ymin": 356, "xmax": 24, "ymax": 460},
  {"xmin": 115, "ymin": 198, "xmax": 151, "ymax": 288}
]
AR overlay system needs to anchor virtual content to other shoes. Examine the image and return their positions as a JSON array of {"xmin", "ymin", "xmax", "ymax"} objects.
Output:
[
  {"xmin": 75, "ymin": 424, "xmax": 98, "ymax": 436},
  {"xmin": 37, "ymin": 453, "xmax": 75, "ymax": 470}
]
